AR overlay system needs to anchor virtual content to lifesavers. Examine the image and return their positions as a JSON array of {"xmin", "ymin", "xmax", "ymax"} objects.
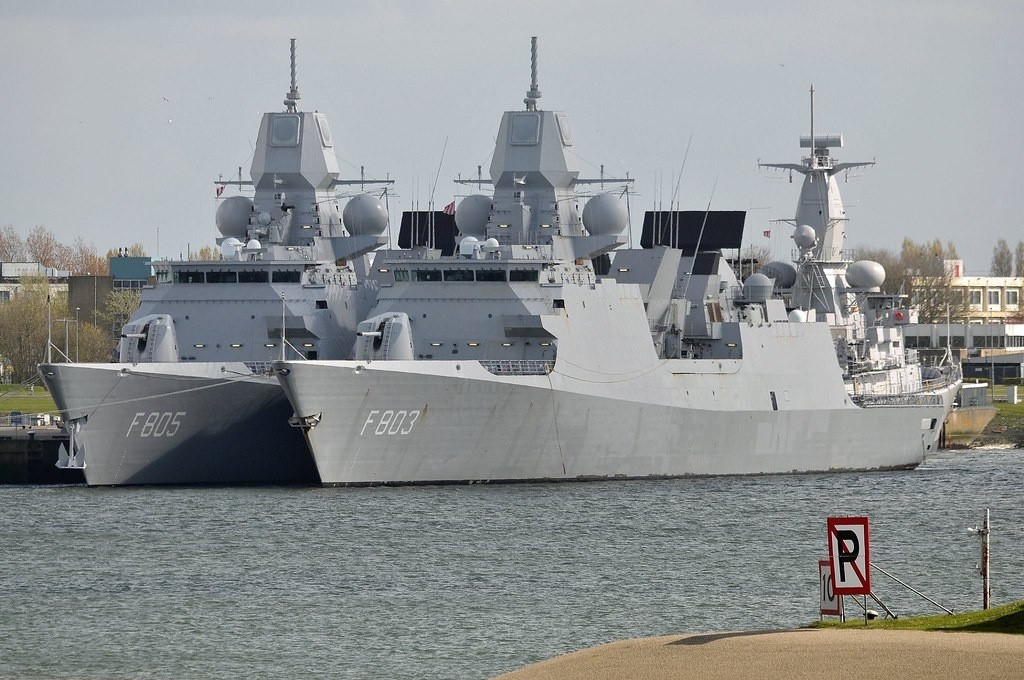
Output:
[{"xmin": 894, "ymin": 311, "xmax": 903, "ymax": 321}]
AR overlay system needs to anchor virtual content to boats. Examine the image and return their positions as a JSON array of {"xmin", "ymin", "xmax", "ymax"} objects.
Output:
[
  {"xmin": 35, "ymin": 37, "xmax": 353, "ymax": 487},
  {"xmin": 938, "ymin": 379, "xmax": 1000, "ymax": 451},
  {"xmin": 272, "ymin": 36, "xmax": 966, "ymax": 486}
]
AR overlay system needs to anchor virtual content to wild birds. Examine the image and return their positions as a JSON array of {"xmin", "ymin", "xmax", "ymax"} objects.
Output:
[
  {"xmin": 208, "ymin": 96, "xmax": 216, "ymax": 101},
  {"xmin": 162, "ymin": 96, "xmax": 170, "ymax": 102}
]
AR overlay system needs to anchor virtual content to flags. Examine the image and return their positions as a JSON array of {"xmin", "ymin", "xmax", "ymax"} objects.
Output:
[
  {"xmin": 762, "ymin": 229, "xmax": 771, "ymax": 238},
  {"xmin": 216, "ymin": 186, "xmax": 224, "ymax": 195},
  {"xmin": 443, "ymin": 201, "xmax": 455, "ymax": 214}
]
